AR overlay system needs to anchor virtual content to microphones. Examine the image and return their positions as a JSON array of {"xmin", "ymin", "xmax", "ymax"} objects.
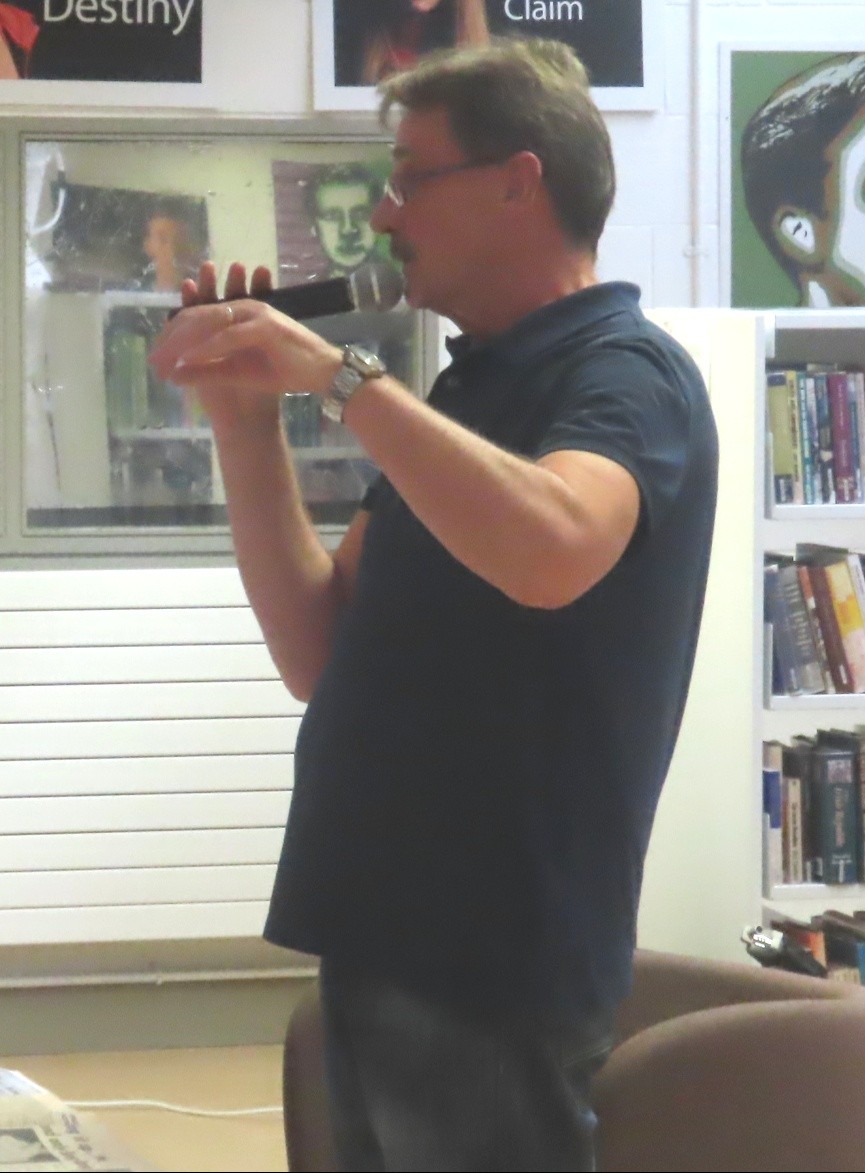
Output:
[{"xmin": 165, "ymin": 261, "xmax": 403, "ymax": 323}]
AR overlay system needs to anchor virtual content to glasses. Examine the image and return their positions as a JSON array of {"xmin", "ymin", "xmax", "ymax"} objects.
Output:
[{"xmin": 384, "ymin": 152, "xmax": 498, "ymax": 208}]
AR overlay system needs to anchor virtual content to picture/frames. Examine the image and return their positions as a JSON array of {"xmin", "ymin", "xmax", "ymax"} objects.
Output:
[
  {"xmin": 310, "ymin": 0, "xmax": 665, "ymax": 112},
  {"xmin": 0, "ymin": 102, "xmax": 438, "ymax": 571},
  {"xmin": 690, "ymin": 0, "xmax": 865, "ymax": 310}
]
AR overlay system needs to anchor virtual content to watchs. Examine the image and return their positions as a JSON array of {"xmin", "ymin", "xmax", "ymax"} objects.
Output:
[{"xmin": 320, "ymin": 341, "xmax": 391, "ymax": 424}]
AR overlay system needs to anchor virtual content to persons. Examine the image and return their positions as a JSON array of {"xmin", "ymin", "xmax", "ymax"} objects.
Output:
[{"xmin": 150, "ymin": 35, "xmax": 719, "ymax": 1173}]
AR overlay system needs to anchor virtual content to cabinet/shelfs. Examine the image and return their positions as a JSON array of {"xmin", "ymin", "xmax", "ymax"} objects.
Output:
[{"xmin": 756, "ymin": 307, "xmax": 865, "ymax": 901}]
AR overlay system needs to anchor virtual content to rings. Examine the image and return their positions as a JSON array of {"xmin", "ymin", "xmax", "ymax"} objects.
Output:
[{"xmin": 223, "ymin": 302, "xmax": 238, "ymax": 328}]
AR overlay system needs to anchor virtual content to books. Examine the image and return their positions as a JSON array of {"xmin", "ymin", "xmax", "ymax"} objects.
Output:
[{"xmin": 758, "ymin": 360, "xmax": 865, "ymax": 898}]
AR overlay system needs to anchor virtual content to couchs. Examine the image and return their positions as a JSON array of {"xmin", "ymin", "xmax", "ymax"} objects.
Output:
[{"xmin": 283, "ymin": 947, "xmax": 865, "ymax": 1172}]
